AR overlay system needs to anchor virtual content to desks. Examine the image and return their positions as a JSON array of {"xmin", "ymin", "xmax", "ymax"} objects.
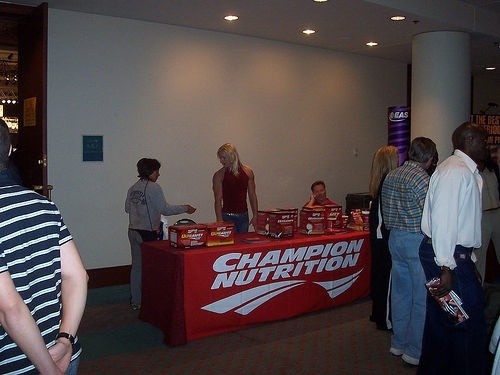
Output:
[{"xmin": 141, "ymin": 230, "xmax": 371, "ymax": 346}]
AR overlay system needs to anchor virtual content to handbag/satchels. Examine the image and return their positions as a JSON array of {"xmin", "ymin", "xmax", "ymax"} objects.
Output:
[{"xmin": 140, "ymin": 222, "xmax": 164, "ymax": 242}]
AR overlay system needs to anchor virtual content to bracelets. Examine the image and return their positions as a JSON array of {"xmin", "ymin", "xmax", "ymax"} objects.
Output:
[
  {"xmin": 56, "ymin": 332, "xmax": 74, "ymax": 346},
  {"xmin": 441, "ymin": 266, "xmax": 451, "ymax": 272}
]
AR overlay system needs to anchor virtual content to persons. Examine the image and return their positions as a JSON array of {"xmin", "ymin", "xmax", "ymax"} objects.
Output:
[
  {"xmin": 370, "ymin": 122, "xmax": 500, "ymax": 375},
  {"xmin": 124, "ymin": 158, "xmax": 197, "ymax": 310},
  {"xmin": 0, "ymin": 119, "xmax": 89, "ymax": 375},
  {"xmin": 303, "ymin": 181, "xmax": 336, "ymax": 206},
  {"xmin": 213, "ymin": 143, "xmax": 258, "ymax": 232}
]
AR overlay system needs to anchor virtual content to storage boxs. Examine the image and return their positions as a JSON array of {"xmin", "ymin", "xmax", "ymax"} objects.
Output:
[
  {"xmin": 258, "ymin": 204, "xmax": 369, "ymax": 238},
  {"xmin": 169, "ymin": 220, "xmax": 235, "ymax": 250}
]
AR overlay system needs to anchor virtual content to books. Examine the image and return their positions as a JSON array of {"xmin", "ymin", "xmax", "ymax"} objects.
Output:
[{"xmin": 425, "ymin": 277, "xmax": 469, "ymax": 325}]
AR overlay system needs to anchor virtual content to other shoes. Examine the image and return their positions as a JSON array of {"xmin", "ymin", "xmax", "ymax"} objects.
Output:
[
  {"xmin": 390, "ymin": 352, "xmax": 403, "ymax": 361},
  {"xmin": 401, "ymin": 358, "xmax": 418, "ymax": 370}
]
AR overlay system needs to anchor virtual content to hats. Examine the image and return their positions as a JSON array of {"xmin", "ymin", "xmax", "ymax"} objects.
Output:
[{"xmin": 135, "ymin": 157, "xmax": 161, "ymax": 178}]
